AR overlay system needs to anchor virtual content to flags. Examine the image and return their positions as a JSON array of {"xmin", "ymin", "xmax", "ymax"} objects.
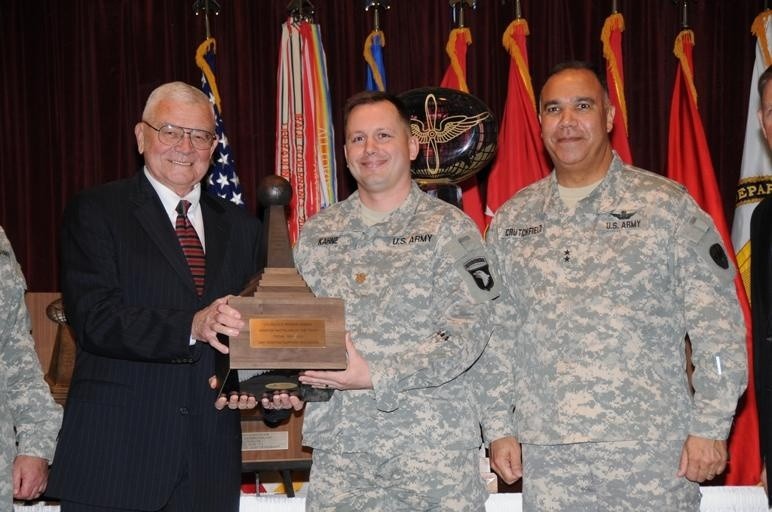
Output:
[
  {"xmin": 485, "ymin": 19, "xmax": 551, "ymax": 236},
  {"xmin": 195, "ymin": 38, "xmax": 244, "ymax": 208},
  {"xmin": 439, "ymin": 27, "xmax": 485, "ymax": 238},
  {"xmin": 272, "ymin": 19, "xmax": 340, "ymax": 249},
  {"xmin": 362, "ymin": 30, "xmax": 391, "ymax": 92},
  {"xmin": 731, "ymin": 9, "xmax": 772, "ymax": 306},
  {"xmin": 601, "ymin": 13, "xmax": 633, "ymax": 167}
]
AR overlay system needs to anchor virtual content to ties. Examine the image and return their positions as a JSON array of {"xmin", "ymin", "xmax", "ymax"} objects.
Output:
[{"xmin": 175, "ymin": 200, "xmax": 206, "ymax": 301}]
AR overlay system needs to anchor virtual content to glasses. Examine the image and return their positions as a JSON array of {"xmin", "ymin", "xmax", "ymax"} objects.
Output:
[{"xmin": 139, "ymin": 121, "xmax": 217, "ymax": 150}]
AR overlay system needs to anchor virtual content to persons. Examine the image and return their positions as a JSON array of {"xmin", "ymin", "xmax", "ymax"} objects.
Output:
[
  {"xmin": 481, "ymin": 62, "xmax": 748, "ymax": 512},
  {"xmin": 750, "ymin": 65, "xmax": 772, "ymax": 511},
  {"xmin": 44, "ymin": 82, "xmax": 265, "ymax": 512},
  {"xmin": 0, "ymin": 223, "xmax": 64, "ymax": 512},
  {"xmin": 262, "ymin": 91, "xmax": 500, "ymax": 512},
  {"xmin": 667, "ymin": 29, "xmax": 764, "ymax": 486}
]
